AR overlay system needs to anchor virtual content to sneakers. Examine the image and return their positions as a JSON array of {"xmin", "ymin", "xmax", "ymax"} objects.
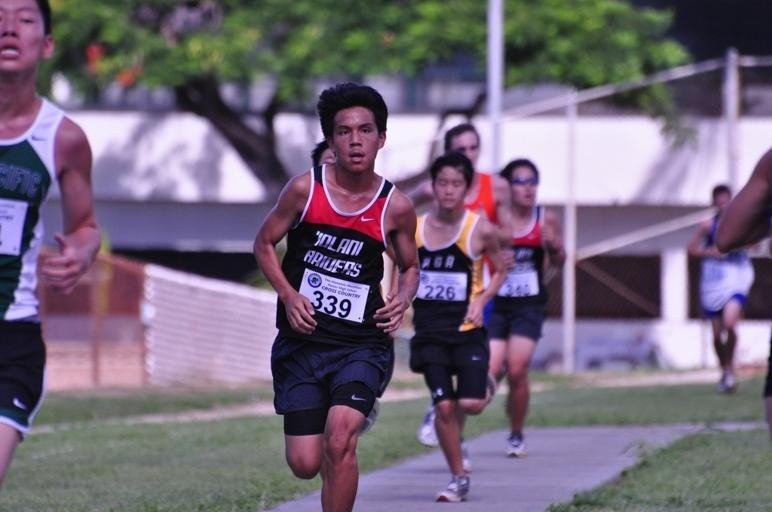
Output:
[
  {"xmin": 362, "ymin": 398, "xmax": 381, "ymax": 433},
  {"xmin": 486, "ymin": 374, "xmax": 497, "ymax": 396},
  {"xmin": 460, "ymin": 439, "xmax": 472, "ymax": 473},
  {"xmin": 435, "ymin": 474, "xmax": 471, "ymax": 502},
  {"xmin": 505, "ymin": 434, "xmax": 526, "ymax": 458},
  {"xmin": 417, "ymin": 405, "xmax": 440, "ymax": 447},
  {"xmin": 716, "ymin": 371, "xmax": 738, "ymax": 394}
]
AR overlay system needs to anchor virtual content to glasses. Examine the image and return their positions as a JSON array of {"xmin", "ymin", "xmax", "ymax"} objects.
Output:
[{"xmin": 510, "ymin": 176, "xmax": 538, "ymax": 186}]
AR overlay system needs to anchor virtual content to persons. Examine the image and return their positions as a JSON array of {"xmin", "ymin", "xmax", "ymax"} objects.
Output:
[
  {"xmin": 490, "ymin": 158, "xmax": 566, "ymax": 460},
  {"xmin": 714, "ymin": 147, "xmax": 771, "ymax": 435},
  {"xmin": 252, "ymin": 81, "xmax": 423, "ymax": 512},
  {"xmin": 1, "ymin": 2, "xmax": 104, "ymax": 489},
  {"xmin": 402, "ymin": 125, "xmax": 518, "ymax": 447},
  {"xmin": 386, "ymin": 152, "xmax": 513, "ymax": 507},
  {"xmin": 688, "ymin": 185, "xmax": 764, "ymax": 397},
  {"xmin": 311, "ymin": 138, "xmax": 340, "ymax": 167}
]
